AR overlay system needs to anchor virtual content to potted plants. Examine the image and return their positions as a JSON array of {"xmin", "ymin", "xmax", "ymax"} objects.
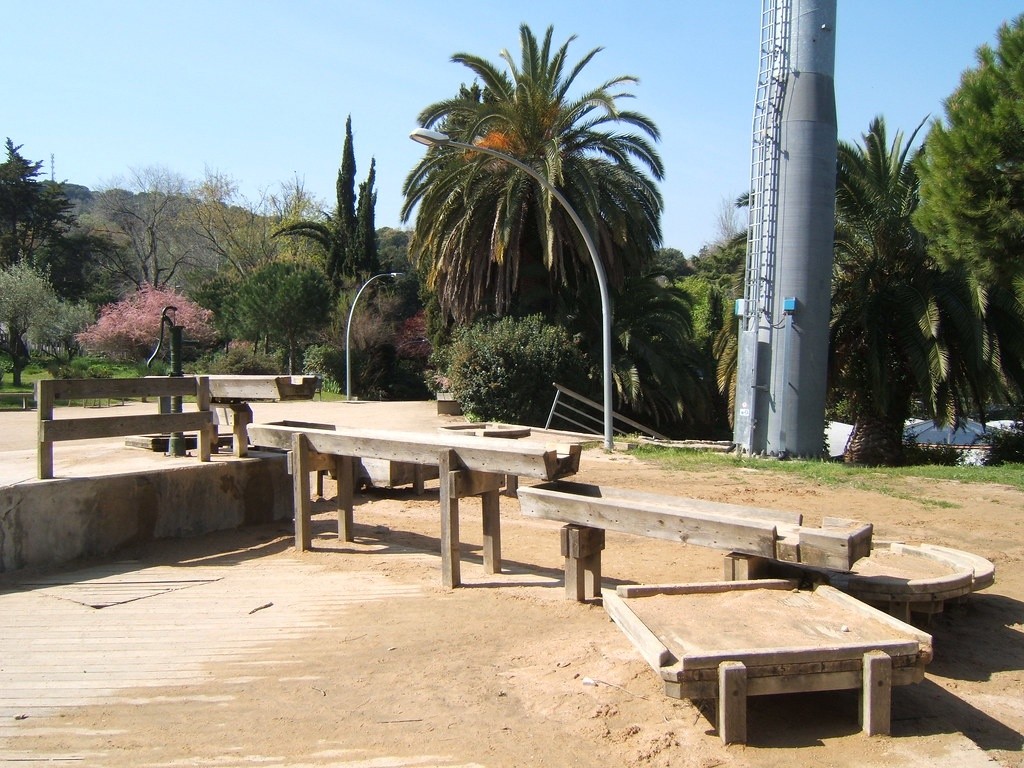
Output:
[{"xmin": 424, "ymin": 371, "xmax": 463, "ymax": 415}]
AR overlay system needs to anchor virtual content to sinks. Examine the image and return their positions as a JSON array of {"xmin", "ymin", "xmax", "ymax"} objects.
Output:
[{"xmin": 143, "ymin": 374, "xmax": 319, "ymax": 400}]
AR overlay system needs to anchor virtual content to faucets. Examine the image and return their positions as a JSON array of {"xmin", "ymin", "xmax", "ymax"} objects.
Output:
[{"xmin": 183, "ymin": 339, "xmax": 201, "ymax": 349}]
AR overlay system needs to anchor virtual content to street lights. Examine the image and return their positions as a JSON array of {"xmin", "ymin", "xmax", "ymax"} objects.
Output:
[
  {"xmin": 345, "ymin": 272, "xmax": 406, "ymax": 402},
  {"xmin": 406, "ymin": 126, "xmax": 617, "ymax": 453}
]
